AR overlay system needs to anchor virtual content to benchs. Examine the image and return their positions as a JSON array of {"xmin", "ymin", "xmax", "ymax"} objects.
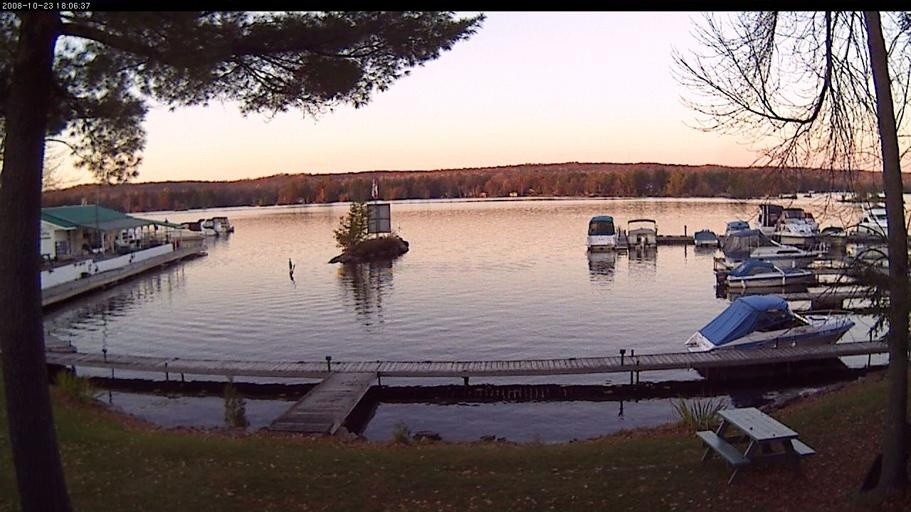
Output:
[
  {"xmin": 697, "ymin": 429, "xmax": 753, "ymax": 484},
  {"xmin": 782, "ymin": 438, "xmax": 817, "ymax": 477}
]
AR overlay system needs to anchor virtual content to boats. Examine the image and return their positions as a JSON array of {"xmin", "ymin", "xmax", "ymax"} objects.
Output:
[
  {"xmin": 586, "ymin": 214, "xmax": 658, "ymax": 250},
  {"xmin": 684, "ymin": 202, "xmax": 887, "ymax": 354}
]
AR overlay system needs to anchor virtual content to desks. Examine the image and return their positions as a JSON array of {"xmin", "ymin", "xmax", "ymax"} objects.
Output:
[{"xmin": 701, "ymin": 406, "xmax": 802, "ymax": 486}]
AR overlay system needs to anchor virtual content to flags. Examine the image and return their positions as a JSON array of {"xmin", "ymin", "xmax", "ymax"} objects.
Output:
[{"xmin": 370, "ymin": 170, "xmax": 380, "ymax": 200}]
